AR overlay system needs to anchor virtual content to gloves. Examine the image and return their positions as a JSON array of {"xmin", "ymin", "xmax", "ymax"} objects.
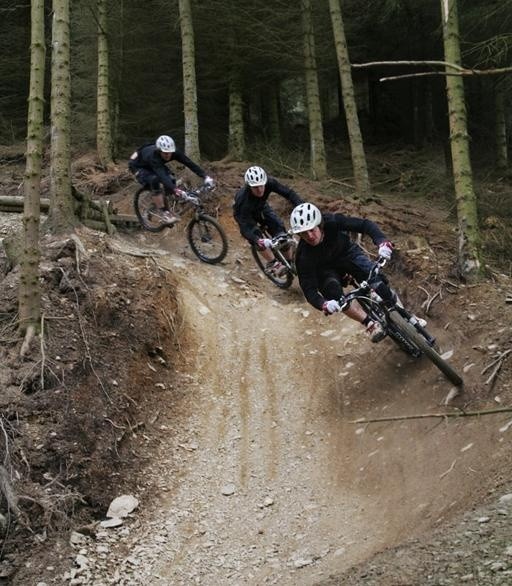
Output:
[
  {"xmin": 257, "ymin": 239, "xmax": 273, "ymax": 249},
  {"xmin": 204, "ymin": 175, "xmax": 214, "ymax": 187},
  {"xmin": 378, "ymin": 240, "xmax": 395, "ymax": 261},
  {"xmin": 174, "ymin": 188, "xmax": 187, "ymax": 198},
  {"xmin": 322, "ymin": 300, "xmax": 342, "ymax": 316}
]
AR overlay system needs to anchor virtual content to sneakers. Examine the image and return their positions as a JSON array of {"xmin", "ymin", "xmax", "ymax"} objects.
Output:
[
  {"xmin": 409, "ymin": 314, "xmax": 427, "ymax": 328},
  {"xmin": 162, "ymin": 210, "xmax": 181, "ymax": 224},
  {"xmin": 366, "ymin": 321, "xmax": 384, "ymax": 343},
  {"xmin": 272, "ymin": 261, "xmax": 289, "ymax": 275}
]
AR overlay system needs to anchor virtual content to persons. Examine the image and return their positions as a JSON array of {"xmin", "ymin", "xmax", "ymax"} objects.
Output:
[
  {"xmin": 128, "ymin": 135, "xmax": 214, "ymax": 224},
  {"xmin": 289, "ymin": 202, "xmax": 428, "ymax": 343},
  {"xmin": 233, "ymin": 166, "xmax": 306, "ymax": 277}
]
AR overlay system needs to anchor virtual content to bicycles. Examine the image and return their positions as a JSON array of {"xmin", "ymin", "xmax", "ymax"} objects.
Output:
[
  {"xmin": 250, "ymin": 230, "xmax": 301, "ymax": 288},
  {"xmin": 323, "ymin": 242, "xmax": 461, "ymax": 389},
  {"xmin": 133, "ymin": 184, "xmax": 228, "ymax": 263}
]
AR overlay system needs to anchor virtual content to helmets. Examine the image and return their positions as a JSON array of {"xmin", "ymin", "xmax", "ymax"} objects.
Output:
[
  {"xmin": 244, "ymin": 166, "xmax": 268, "ymax": 187},
  {"xmin": 290, "ymin": 203, "xmax": 322, "ymax": 234},
  {"xmin": 156, "ymin": 135, "xmax": 176, "ymax": 153}
]
